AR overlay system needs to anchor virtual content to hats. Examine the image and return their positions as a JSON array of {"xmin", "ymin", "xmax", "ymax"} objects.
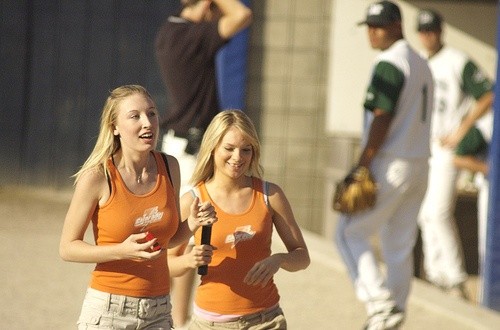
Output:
[
  {"xmin": 417, "ymin": 9, "xmax": 441, "ymax": 32},
  {"xmin": 357, "ymin": 1, "xmax": 400, "ymax": 27}
]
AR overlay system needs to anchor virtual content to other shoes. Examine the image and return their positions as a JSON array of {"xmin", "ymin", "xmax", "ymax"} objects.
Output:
[{"xmin": 362, "ymin": 303, "xmax": 408, "ymax": 330}]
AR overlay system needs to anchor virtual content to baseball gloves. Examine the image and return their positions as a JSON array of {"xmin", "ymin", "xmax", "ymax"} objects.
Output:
[{"xmin": 332, "ymin": 165, "xmax": 377, "ymax": 215}]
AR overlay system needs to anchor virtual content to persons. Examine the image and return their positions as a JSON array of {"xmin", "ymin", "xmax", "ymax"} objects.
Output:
[
  {"xmin": 415, "ymin": 9, "xmax": 496, "ymax": 302},
  {"xmin": 333, "ymin": 0, "xmax": 434, "ymax": 330},
  {"xmin": 59, "ymin": 85, "xmax": 219, "ymax": 330},
  {"xmin": 156, "ymin": 0, "xmax": 252, "ymax": 330},
  {"xmin": 167, "ymin": 108, "xmax": 310, "ymax": 330}
]
